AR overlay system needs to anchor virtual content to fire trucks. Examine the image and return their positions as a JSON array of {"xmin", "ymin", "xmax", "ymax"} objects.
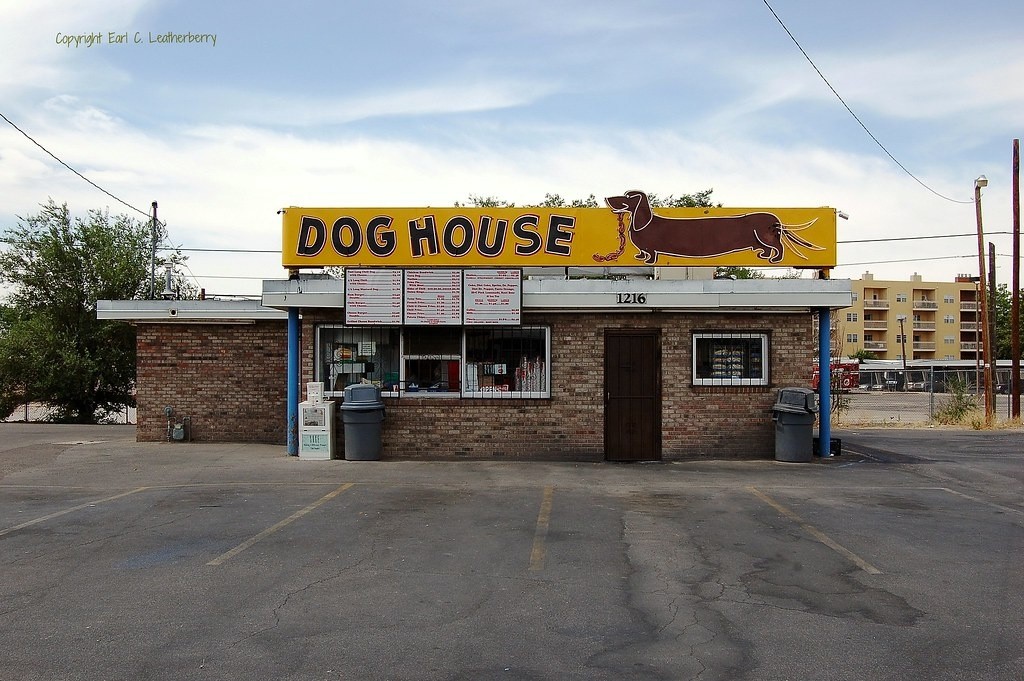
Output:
[{"xmin": 811, "ymin": 357, "xmax": 861, "ymax": 395}]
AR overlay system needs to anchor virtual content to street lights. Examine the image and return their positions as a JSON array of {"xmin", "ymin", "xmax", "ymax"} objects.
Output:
[
  {"xmin": 897, "ymin": 315, "xmax": 907, "ymax": 370},
  {"xmin": 974, "ymin": 173, "xmax": 993, "ymax": 421}
]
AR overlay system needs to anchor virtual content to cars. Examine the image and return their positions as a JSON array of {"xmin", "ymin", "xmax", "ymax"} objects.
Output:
[
  {"xmin": 858, "ymin": 375, "xmax": 941, "ymax": 393},
  {"xmin": 994, "ymin": 378, "xmax": 1024, "ymax": 395}
]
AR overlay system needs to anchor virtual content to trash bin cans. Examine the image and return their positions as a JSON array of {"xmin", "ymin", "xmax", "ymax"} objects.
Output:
[
  {"xmin": 770, "ymin": 387, "xmax": 816, "ymax": 463},
  {"xmin": 340, "ymin": 384, "xmax": 385, "ymax": 461}
]
